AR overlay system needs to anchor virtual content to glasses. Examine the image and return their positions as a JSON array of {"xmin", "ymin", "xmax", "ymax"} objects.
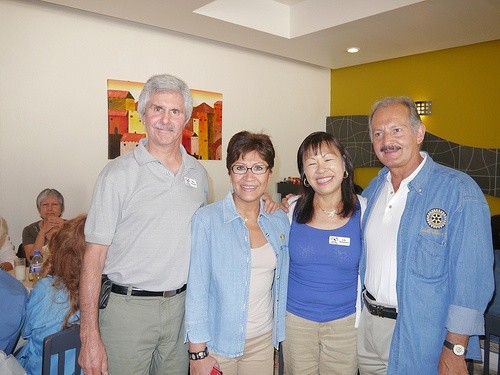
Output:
[{"xmin": 230, "ymin": 163, "xmax": 270, "ymax": 175}]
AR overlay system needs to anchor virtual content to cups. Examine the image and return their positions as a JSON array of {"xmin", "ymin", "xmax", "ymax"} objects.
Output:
[{"xmin": 14, "ymin": 258, "xmax": 26, "ymax": 282}]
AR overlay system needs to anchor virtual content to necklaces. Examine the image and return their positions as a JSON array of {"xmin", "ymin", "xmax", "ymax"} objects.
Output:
[
  {"xmin": 237, "ymin": 208, "xmax": 259, "ymax": 221},
  {"xmin": 314, "ymin": 200, "xmax": 342, "ymax": 218}
]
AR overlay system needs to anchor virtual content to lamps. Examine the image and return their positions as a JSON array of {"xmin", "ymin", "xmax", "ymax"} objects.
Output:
[{"xmin": 415, "ymin": 101, "xmax": 431, "ymax": 115}]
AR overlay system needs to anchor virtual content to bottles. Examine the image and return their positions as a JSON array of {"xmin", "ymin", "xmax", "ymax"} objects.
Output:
[{"xmin": 30, "ymin": 250, "xmax": 45, "ymax": 289}]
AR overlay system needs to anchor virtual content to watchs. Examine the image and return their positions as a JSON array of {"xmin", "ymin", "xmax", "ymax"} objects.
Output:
[{"xmin": 443, "ymin": 339, "xmax": 468, "ymax": 356}]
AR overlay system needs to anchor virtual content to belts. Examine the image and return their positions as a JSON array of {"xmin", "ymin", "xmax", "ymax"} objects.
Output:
[
  {"xmin": 362, "ymin": 293, "xmax": 398, "ymax": 320},
  {"xmin": 102, "ymin": 274, "xmax": 187, "ymax": 298}
]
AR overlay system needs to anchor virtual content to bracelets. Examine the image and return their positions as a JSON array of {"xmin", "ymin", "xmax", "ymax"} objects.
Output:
[{"xmin": 188, "ymin": 346, "xmax": 210, "ymax": 360}]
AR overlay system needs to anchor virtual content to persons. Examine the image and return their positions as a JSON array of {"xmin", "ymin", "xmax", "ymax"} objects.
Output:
[
  {"xmin": 0, "ymin": 187, "xmax": 87, "ymax": 375},
  {"xmin": 79, "ymin": 74, "xmax": 281, "ymax": 375},
  {"xmin": 286, "ymin": 131, "xmax": 368, "ymax": 375},
  {"xmin": 184, "ymin": 130, "xmax": 290, "ymax": 375},
  {"xmin": 281, "ymin": 96, "xmax": 495, "ymax": 375}
]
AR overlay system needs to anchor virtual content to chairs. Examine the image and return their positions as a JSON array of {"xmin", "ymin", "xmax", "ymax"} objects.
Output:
[
  {"xmin": 468, "ymin": 313, "xmax": 500, "ymax": 375},
  {"xmin": 42, "ymin": 324, "xmax": 83, "ymax": 375}
]
